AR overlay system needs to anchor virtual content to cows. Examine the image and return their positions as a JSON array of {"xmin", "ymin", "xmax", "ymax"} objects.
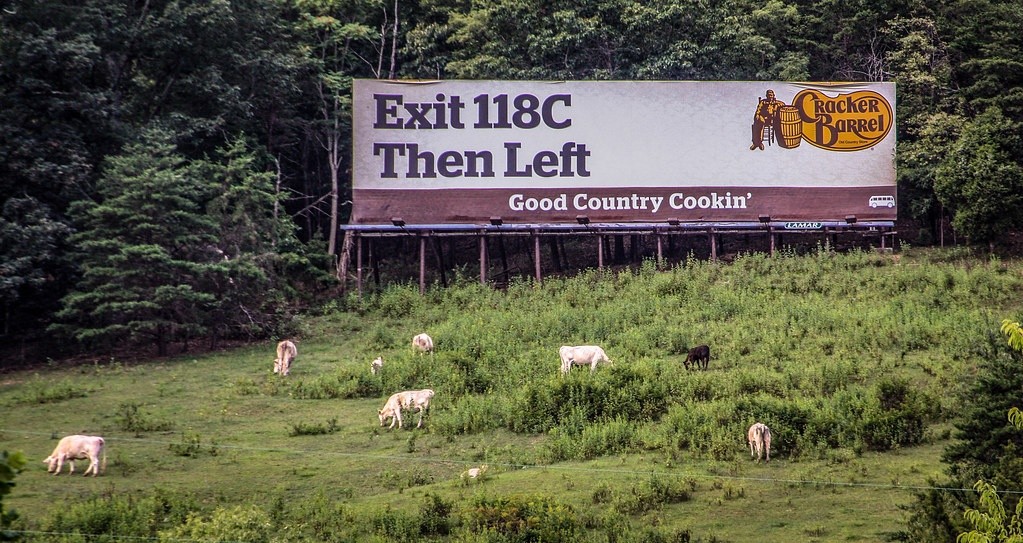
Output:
[
  {"xmin": 371, "ymin": 331, "xmax": 435, "ymax": 433},
  {"xmin": 558, "ymin": 346, "xmax": 614, "ymax": 377},
  {"xmin": 748, "ymin": 421, "xmax": 771, "ymax": 463},
  {"xmin": 273, "ymin": 341, "xmax": 296, "ymax": 378},
  {"xmin": 682, "ymin": 345, "xmax": 709, "ymax": 371},
  {"xmin": 42, "ymin": 435, "xmax": 105, "ymax": 479}
]
